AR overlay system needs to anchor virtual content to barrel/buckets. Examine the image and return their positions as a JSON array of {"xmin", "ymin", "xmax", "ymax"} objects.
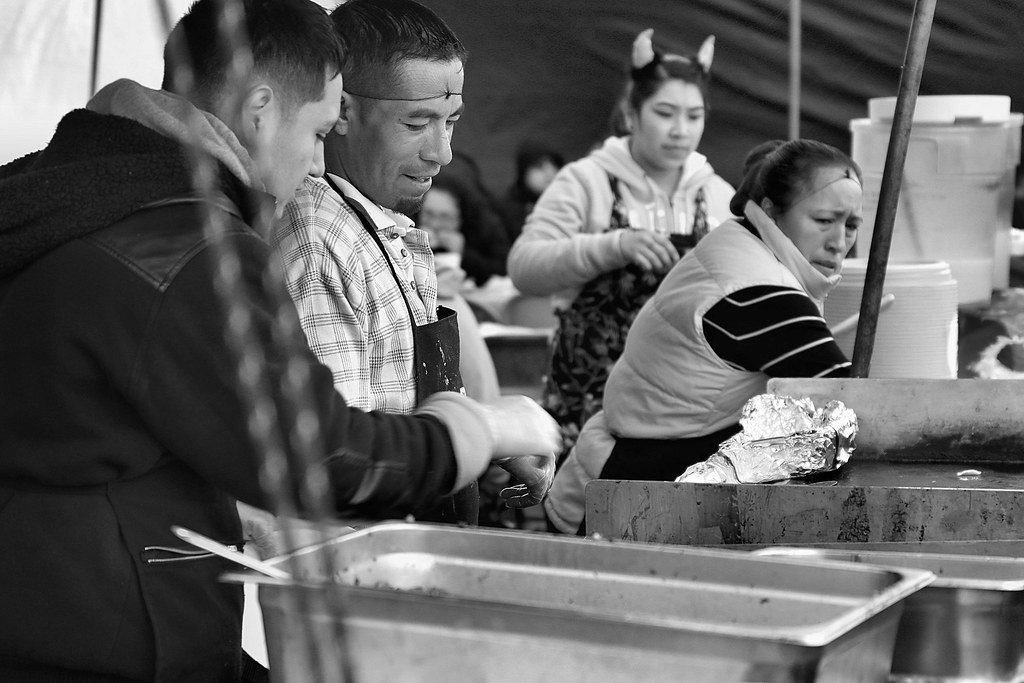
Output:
[
  {"xmin": 823, "ymin": 256, "xmax": 959, "ymax": 380},
  {"xmin": 850, "ymin": 94, "xmax": 1024, "ymax": 302}
]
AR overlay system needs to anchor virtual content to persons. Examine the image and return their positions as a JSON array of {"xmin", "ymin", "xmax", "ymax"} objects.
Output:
[
  {"xmin": 418, "ymin": 157, "xmax": 520, "ymax": 327},
  {"xmin": 275, "ymin": 0, "xmax": 554, "ymax": 535},
  {"xmin": 508, "ymin": 29, "xmax": 749, "ymax": 479},
  {"xmin": 499, "ymin": 138, "xmax": 568, "ymax": 246},
  {"xmin": 0, "ymin": 0, "xmax": 562, "ymax": 683},
  {"xmin": 543, "ymin": 140, "xmax": 866, "ymax": 540}
]
